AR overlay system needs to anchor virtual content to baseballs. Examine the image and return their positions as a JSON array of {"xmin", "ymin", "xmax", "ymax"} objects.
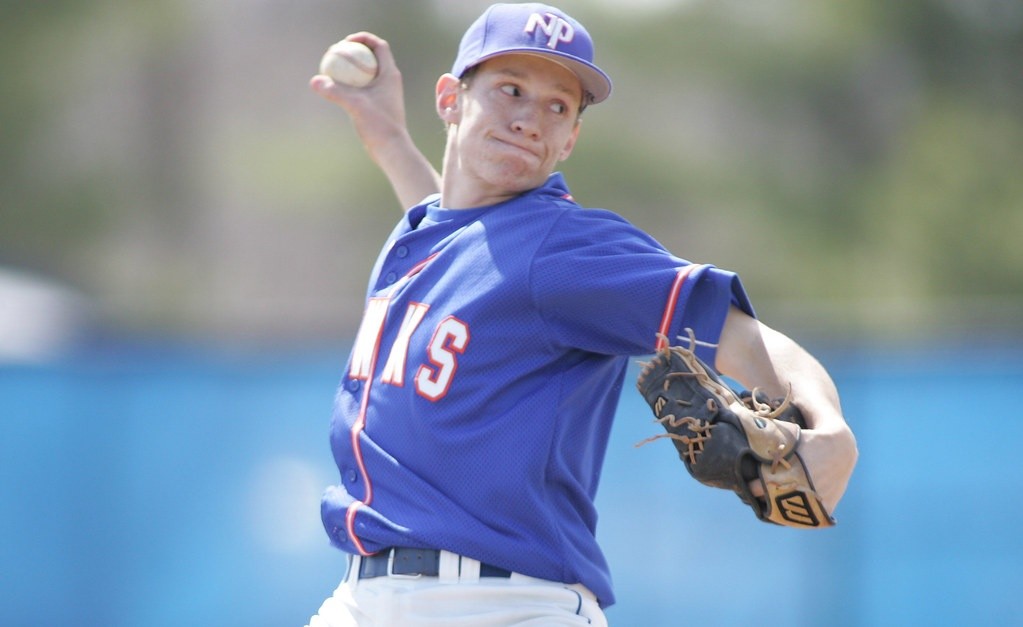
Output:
[{"xmin": 320, "ymin": 39, "xmax": 377, "ymax": 89}]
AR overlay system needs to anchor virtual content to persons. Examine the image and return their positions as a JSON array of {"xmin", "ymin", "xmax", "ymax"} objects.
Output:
[{"xmin": 302, "ymin": 3, "xmax": 858, "ymax": 627}]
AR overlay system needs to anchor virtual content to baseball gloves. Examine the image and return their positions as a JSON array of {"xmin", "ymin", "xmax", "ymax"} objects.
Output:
[{"xmin": 637, "ymin": 344, "xmax": 838, "ymax": 530}]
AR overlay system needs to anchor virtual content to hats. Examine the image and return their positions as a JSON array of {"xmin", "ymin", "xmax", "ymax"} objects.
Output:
[{"xmin": 451, "ymin": 2, "xmax": 612, "ymax": 114}]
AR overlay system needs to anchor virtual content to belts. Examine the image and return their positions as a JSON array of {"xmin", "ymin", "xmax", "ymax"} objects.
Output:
[{"xmin": 344, "ymin": 547, "xmax": 510, "ymax": 578}]
